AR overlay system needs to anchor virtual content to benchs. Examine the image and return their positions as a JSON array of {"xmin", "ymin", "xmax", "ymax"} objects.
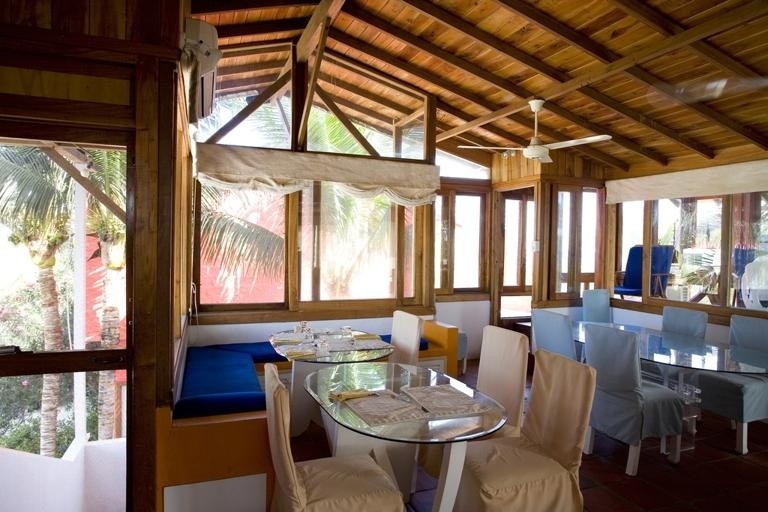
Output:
[{"xmin": 155, "ymin": 318, "xmax": 460, "ymax": 511}]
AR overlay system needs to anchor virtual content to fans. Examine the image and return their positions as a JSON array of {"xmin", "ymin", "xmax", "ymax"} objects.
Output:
[{"xmin": 456, "ymin": 98, "xmax": 614, "ymax": 164}]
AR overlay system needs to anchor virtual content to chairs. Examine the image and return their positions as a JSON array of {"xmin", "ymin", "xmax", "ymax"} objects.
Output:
[
  {"xmin": 695, "ymin": 313, "xmax": 766, "ymax": 453},
  {"xmin": 457, "ymin": 350, "xmax": 595, "ymax": 511},
  {"xmin": 530, "ymin": 309, "xmax": 576, "ymax": 364},
  {"xmin": 581, "ymin": 289, "xmax": 613, "ymax": 318},
  {"xmin": 390, "ymin": 309, "xmax": 422, "ymax": 359},
  {"xmin": 613, "ymin": 245, "xmax": 675, "ymax": 300},
  {"xmin": 732, "ymin": 249, "xmax": 768, "ymax": 308},
  {"xmin": 263, "ymin": 361, "xmax": 406, "ymax": 512},
  {"xmin": 416, "ymin": 326, "xmax": 527, "ymax": 480},
  {"xmin": 584, "ymin": 325, "xmax": 688, "ymax": 475},
  {"xmin": 641, "ymin": 304, "xmax": 707, "ymax": 387}
]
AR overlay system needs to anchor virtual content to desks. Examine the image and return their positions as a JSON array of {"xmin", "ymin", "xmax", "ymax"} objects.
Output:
[
  {"xmin": 303, "ymin": 359, "xmax": 510, "ymax": 511},
  {"xmin": 268, "ymin": 326, "xmax": 394, "ymax": 440},
  {"xmin": 519, "ymin": 319, "xmax": 766, "ymax": 457}
]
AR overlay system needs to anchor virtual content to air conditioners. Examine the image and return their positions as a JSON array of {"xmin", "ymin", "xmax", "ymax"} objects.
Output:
[{"xmin": 184, "ymin": 17, "xmax": 219, "ymax": 119}]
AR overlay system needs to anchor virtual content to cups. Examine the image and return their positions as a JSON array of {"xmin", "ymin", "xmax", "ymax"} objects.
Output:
[{"xmin": 295, "ymin": 320, "xmax": 353, "ymax": 358}]
